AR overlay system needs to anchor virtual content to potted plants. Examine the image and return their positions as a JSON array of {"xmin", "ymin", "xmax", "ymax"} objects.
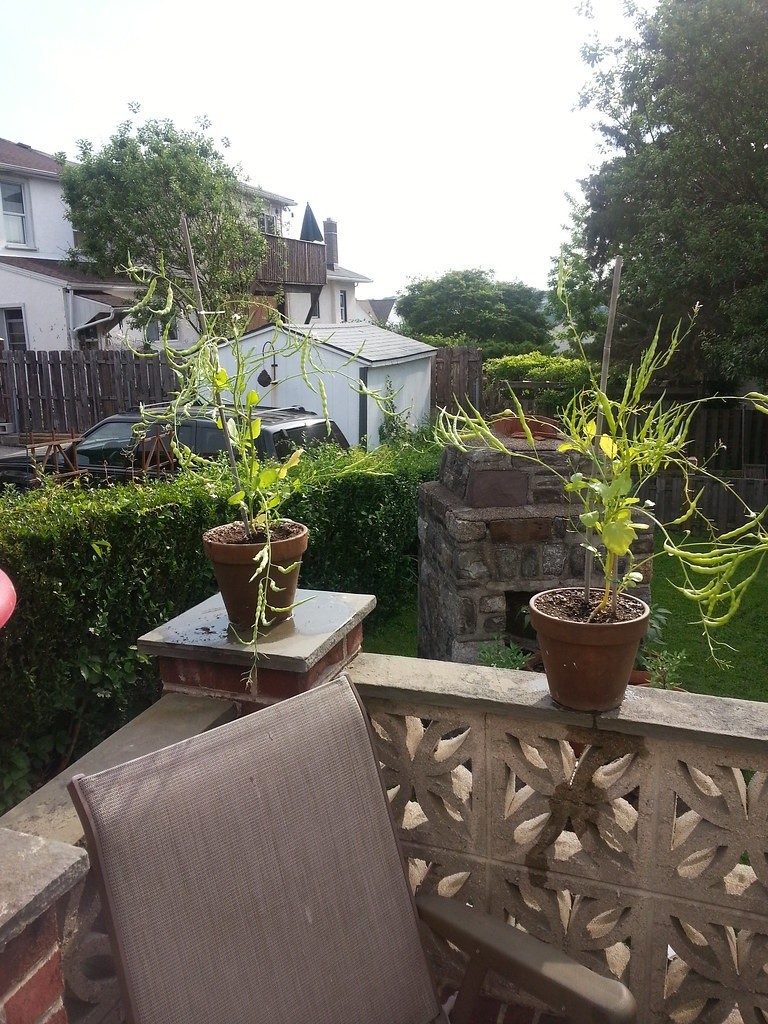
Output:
[
  {"xmin": 435, "ymin": 271, "xmax": 768, "ymax": 713},
  {"xmin": 103, "ymin": 262, "xmax": 398, "ymax": 695}
]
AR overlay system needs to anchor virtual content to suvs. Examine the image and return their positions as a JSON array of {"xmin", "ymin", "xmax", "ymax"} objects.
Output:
[{"xmin": 1, "ymin": 402, "xmax": 348, "ymax": 491}]
